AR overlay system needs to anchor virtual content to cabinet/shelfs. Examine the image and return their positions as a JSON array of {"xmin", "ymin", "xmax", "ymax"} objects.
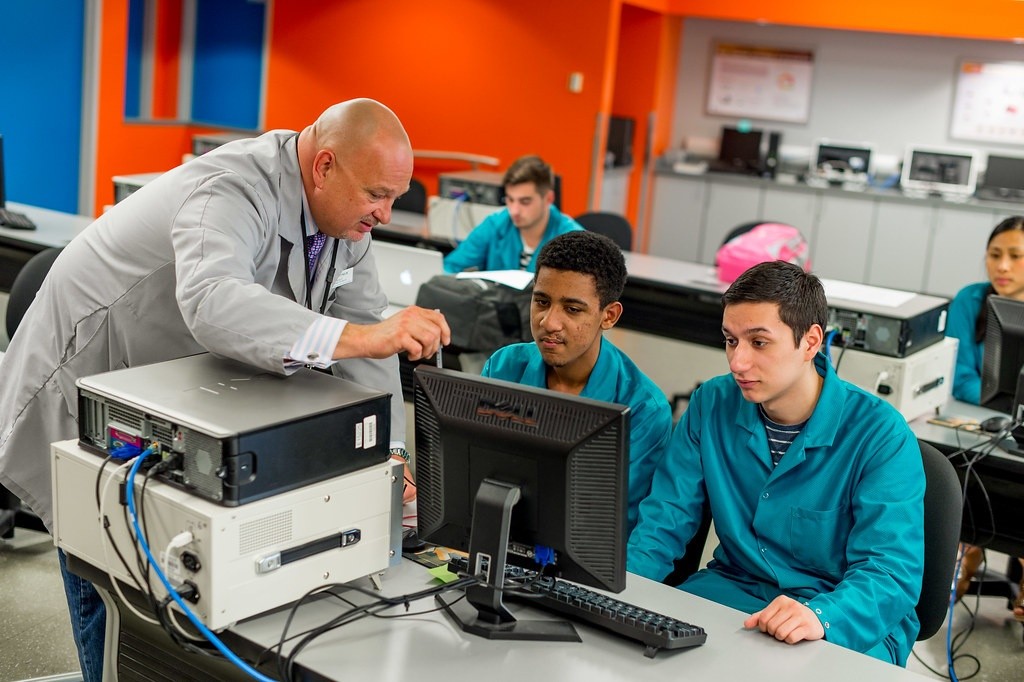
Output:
[{"xmin": 647, "ymin": 161, "xmax": 1024, "ymax": 300}]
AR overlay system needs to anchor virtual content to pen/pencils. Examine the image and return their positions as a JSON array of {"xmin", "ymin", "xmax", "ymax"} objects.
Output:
[{"xmin": 434, "ymin": 308, "xmax": 443, "ymax": 369}]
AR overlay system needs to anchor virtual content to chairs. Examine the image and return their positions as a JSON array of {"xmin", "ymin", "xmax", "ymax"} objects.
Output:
[
  {"xmin": 913, "ymin": 436, "xmax": 967, "ymax": 641},
  {"xmin": 392, "ymin": 176, "xmax": 427, "ymax": 212},
  {"xmin": 5, "ymin": 245, "xmax": 66, "ymax": 342},
  {"xmin": 572, "ymin": 211, "xmax": 633, "ymax": 252},
  {"xmin": 661, "ymin": 504, "xmax": 713, "ymax": 586}
]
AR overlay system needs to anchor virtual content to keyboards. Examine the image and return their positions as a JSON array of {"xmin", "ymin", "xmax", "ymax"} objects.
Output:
[
  {"xmin": 447, "ymin": 554, "xmax": 707, "ymax": 659},
  {"xmin": 0, "ymin": 207, "xmax": 36, "ymax": 231}
]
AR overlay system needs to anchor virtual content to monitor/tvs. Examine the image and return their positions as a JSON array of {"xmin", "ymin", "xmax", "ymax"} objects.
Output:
[
  {"xmin": 408, "ymin": 364, "xmax": 631, "ymax": 640},
  {"xmin": 718, "ymin": 125, "xmax": 761, "ymax": 168},
  {"xmin": 811, "ymin": 138, "xmax": 873, "ymax": 185},
  {"xmin": 900, "ymin": 147, "xmax": 978, "ymax": 203},
  {"xmin": 981, "ymin": 294, "xmax": 1024, "ymax": 458},
  {"xmin": 989, "ymin": 155, "xmax": 1024, "ymax": 199}
]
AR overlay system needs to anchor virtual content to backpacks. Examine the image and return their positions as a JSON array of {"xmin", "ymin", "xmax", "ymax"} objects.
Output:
[
  {"xmin": 716, "ymin": 223, "xmax": 811, "ymax": 282},
  {"xmin": 416, "ymin": 275, "xmax": 539, "ymax": 353}
]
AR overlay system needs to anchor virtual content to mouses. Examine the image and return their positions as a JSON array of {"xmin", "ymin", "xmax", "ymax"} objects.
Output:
[
  {"xmin": 980, "ymin": 416, "xmax": 1012, "ymax": 434},
  {"xmin": 400, "ymin": 526, "xmax": 429, "ymax": 554}
]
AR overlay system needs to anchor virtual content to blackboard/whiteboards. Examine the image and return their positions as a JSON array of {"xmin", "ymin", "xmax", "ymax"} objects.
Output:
[
  {"xmin": 703, "ymin": 42, "xmax": 813, "ymax": 126},
  {"xmin": 948, "ymin": 53, "xmax": 1024, "ymax": 148}
]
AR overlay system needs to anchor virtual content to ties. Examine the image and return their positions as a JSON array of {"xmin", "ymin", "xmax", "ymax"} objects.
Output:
[{"xmin": 303, "ymin": 232, "xmax": 329, "ymax": 286}]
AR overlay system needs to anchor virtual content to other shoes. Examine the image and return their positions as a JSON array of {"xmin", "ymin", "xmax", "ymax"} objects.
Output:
[
  {"xmin": 1012, "ymin": 570, "xmax": 1024, "ymax": 621},
  {"xmin": 952, "ymin": 550, "xmax": 986, "ymax": 604}
]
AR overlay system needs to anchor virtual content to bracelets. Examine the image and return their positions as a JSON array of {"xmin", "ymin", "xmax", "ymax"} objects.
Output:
[{"xmin": 390, "ymin": 448, "xmax": 410, "ymax": 461}]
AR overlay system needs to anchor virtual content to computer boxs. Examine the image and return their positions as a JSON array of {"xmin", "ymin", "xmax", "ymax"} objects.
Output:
[
  {"xmin": 438, "ymin": 168, "xmax": 508, "ymax": 207},
  {"xmin": 817, "ymin": 278, "xmax": 953, "ymax": 359},
  {"xmin": 75, "ymin": 350, "xmax": 393, "ymax": 509}
]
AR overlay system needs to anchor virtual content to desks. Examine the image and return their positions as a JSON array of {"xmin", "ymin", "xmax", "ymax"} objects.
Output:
[
  {"xmin": 113, "ymin": 168, "xmax": 501, "ymax": 248},
  {"xmin": 903, "ymin": 398, "xmax": 1024, "ymax": 557},
  {"xmin": 370, "ymin": 212, "xmax": 466, "ymax": 250},
  {"xmin": 60, "ymin": 545, "xmax": 946, "ymax": 682},
  {"xmin": 0, "ymin": 204, "xmax": 96, "ymax": 248}
]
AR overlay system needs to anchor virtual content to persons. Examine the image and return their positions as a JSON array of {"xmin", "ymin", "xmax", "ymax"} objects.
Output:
[
  {"xmin": 482, "ymin": 230, "xmax": 672, "ymax": 534},
  {"xmin": 627, "ymin": 260, "xmax": 925, "ymax": 668},
  {"xmin": 946, "ymin": 216, "xmax": 1024, "ymax": 615},
  {"xmin": 0, "ymin": 98, "xmax": 451, "ymax": 682},
  {"xmin": 443, "ymin": 155, "xmax": 585, "ymax": 273}
]
